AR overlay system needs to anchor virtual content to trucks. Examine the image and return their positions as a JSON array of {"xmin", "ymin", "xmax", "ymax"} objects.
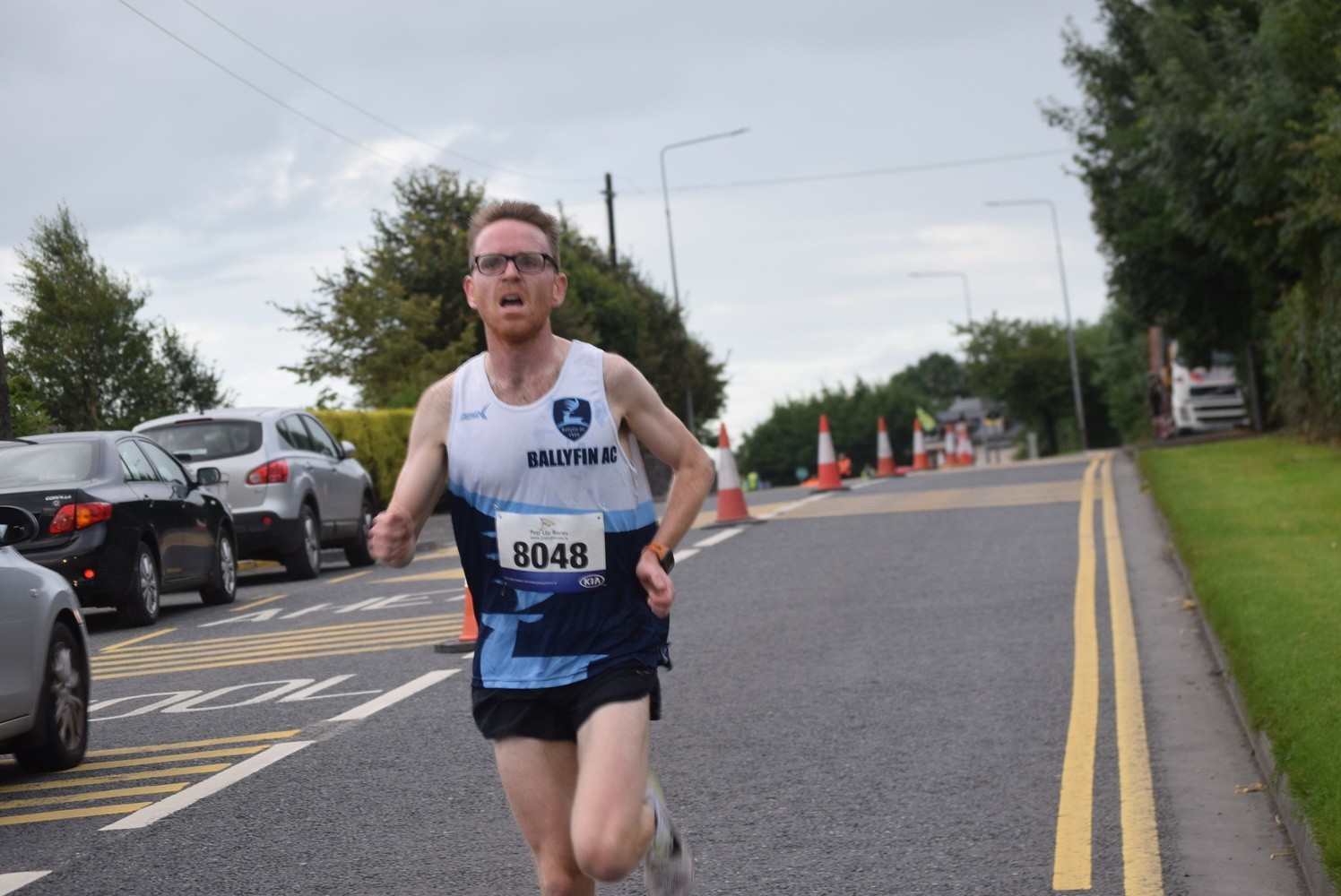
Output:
[{"xmin": 1165, "ymin": 340, "xmax": 1251, "ymax": 434}]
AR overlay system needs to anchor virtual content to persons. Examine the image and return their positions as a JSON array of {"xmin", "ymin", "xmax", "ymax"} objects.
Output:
[
  {"xmin": 836, "ymin": 453, "xmax": 853, "ymax": 478},
  {"xmin": 364, "ymin": 200, "xmax": 714, "ymax": 896},
  {"xmin": 747, "ymin": 469, "xmax": 759, "ymax": 491}
]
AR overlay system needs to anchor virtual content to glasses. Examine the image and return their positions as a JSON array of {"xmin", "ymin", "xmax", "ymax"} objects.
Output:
[{"xmin": 469, "ymin": 251, "xmax": 562, "ymax": 274}]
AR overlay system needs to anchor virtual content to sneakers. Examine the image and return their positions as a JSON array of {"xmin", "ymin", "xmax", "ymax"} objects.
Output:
[{"xmin": 641, "ymin": 775, "xmax": 693, "ymax": 895}]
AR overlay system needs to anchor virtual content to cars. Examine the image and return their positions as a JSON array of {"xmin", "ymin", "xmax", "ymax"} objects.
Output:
[
  {"xmin": 0, "ymin": 504, "xmax": 93, "ymax": 776},
  {"xmin": 0, "ymin": 429, "xmax": 240, "ymax": 629}
]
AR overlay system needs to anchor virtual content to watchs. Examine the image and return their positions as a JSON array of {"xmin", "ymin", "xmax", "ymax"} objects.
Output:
[{"xmin": 641, "ymin": 543, "xmax": 675, "ymax": 576}]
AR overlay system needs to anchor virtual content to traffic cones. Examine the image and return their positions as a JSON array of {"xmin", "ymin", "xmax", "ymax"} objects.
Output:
[
  {"xmin": 904, "ymin": 416, "xmax": 940, "ymax": 472},
  {"xmin": 869, "ymin": 415, "xmax": 907, "ymax": 481},
  {"xmin": 433, "ymin": 579, "xmax": 478, "ymax": 653},
  {"xmin": 694, "ymin": 422, "xmax": 768, "ymax": 530},
  {"xmin": 808, "ymin": 414, "xmax": 850, "ymax": 495},
  {"xmin": 937, "ymin": 420, "xmax": 978, "ymax": 471}
]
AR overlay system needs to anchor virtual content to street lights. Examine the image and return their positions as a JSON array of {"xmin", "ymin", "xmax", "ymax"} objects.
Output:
[
  {"xmin": 658, "ymin": 125, "xmax": 752, "ymax": 441},
  {"xmin": 911, "ymin": 269, "xmax": 991, "ymax": 467},
  {"xmin": 985, "ymin": 195, "xmax": 1090, "ymax": 453}
]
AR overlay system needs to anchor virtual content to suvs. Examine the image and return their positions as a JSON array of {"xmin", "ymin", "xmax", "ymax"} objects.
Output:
[{"xmin": 126, "ymin": 405, "xmax": 382, "ymax": 583}]
